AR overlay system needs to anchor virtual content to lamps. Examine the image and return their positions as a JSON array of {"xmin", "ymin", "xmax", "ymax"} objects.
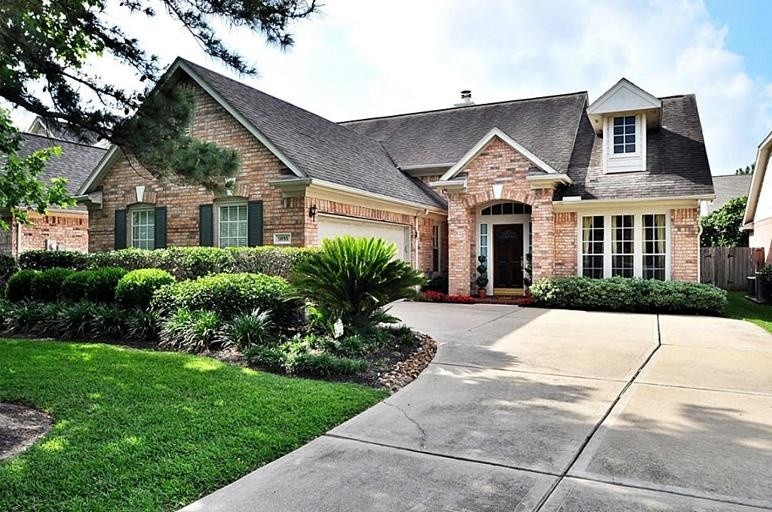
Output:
[{"xmin": 309, "ymin": 205, "xmax": 318, "ymax": 217}]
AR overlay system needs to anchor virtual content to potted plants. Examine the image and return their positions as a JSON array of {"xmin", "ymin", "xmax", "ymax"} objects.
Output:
[
  {"xmin": 523, "ymin": 253, "xmax": 532, "ymax": 298},
  {"xmin": 476, "ymin": 256, "xmax": 488, "ymax": 299}
]
